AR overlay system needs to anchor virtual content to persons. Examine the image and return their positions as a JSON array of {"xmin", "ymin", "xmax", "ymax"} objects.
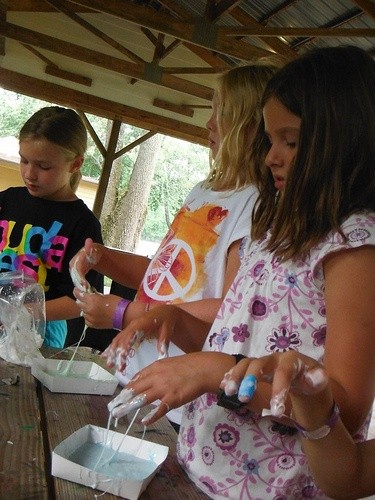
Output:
[
  {"xmin": 0, "ymin": 105, "xmax": 103, "ymax": 346},
  {"xmin": 69, "ymin": 63, "xmax": 286, "ymax": 434},
  {"xmin": 95, "ymin": 45, "xmax": 375, "ymax": 500}
]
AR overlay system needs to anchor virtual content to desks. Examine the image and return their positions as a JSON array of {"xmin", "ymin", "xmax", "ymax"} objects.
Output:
[{"xmin": 0, "ymin": 348, "xmax": 211, "ymax": 500}]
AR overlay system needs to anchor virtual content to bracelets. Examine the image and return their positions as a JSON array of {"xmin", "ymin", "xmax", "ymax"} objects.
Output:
[
  {"xmin": 145, "ymin": 303, "xmax": 150, "ymax": 312},
  {"xmin": 289, "ymin": 400, "xmax": 340, "ymax": 440},
  {"xmin": 114, "ymin": 298, "xmax": 131, "ymax": 331}
]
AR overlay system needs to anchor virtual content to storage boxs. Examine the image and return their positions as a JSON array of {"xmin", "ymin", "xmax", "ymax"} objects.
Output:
[
  {"xmin": 30, "ymin": 358, "xmax": 119, "ymax": 395},
  {"xmin": 50, "ymin": 424, "xmax": 169, "ymax": 500}
]
